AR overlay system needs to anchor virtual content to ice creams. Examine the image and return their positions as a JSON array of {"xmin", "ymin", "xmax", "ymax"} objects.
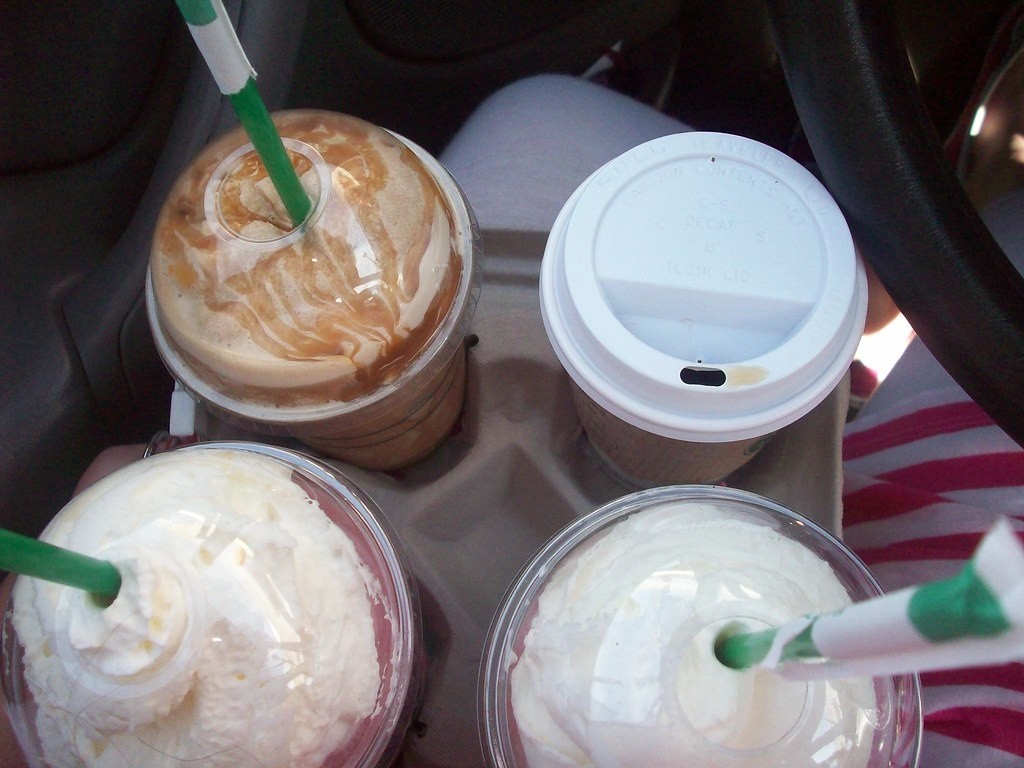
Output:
[
  {"xmin": 146, "ymin": 110, "xmax": 472, "ymax": 470},
  {"xmin": 8, "ymin": 447, "xmax": 384, "ymax": 768},
  {"xmin": 503, "ymin": 502, "xmax": 881, "ymax": 768}
]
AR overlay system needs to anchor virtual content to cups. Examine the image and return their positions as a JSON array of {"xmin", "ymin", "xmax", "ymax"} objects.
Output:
[
  {"xmin": 0, "ymin": 440, "xmax": 424, "ymax": 768},
  {"xmin": 537, "ymin": 126, "xmax": 870, "ymax": 492},
  {"xmin": 475, "ymin": 484, "xmax": 924, "ymax": 768},
  {"xmin": 144, "ymin": 105, "xmax": 484, "ymax": 475}
]
